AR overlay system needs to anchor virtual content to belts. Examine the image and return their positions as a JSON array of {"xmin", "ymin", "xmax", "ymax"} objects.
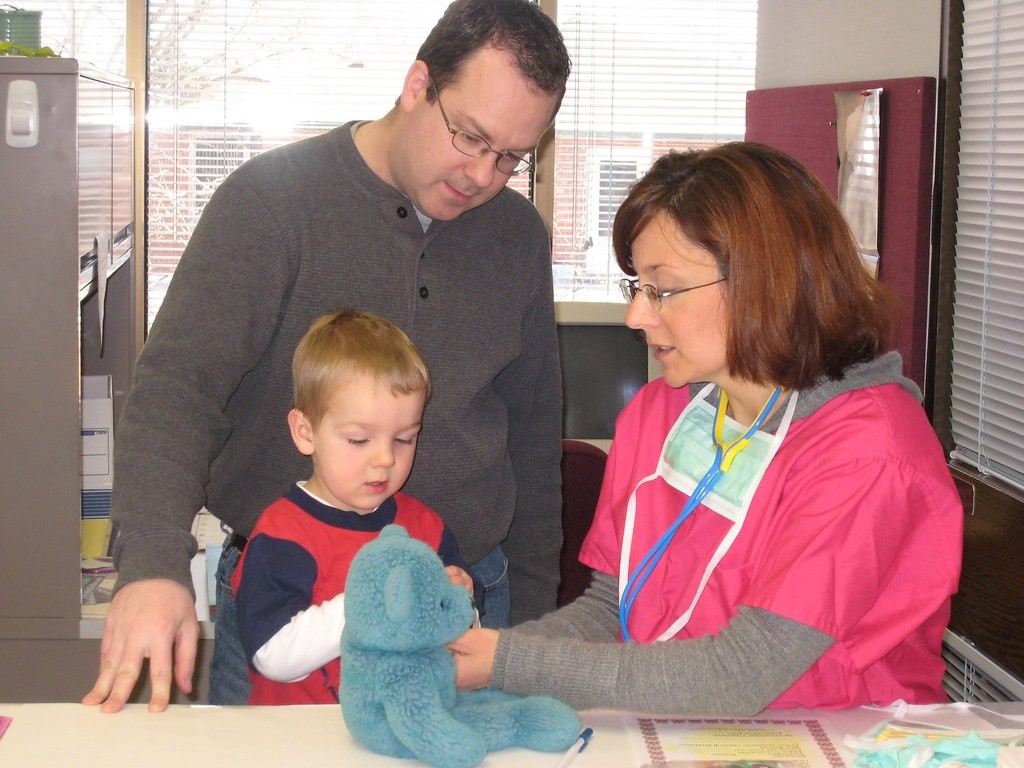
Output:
[{"xmin": 233, "ymin": 534, "xmax": 248, "ymax": 552}]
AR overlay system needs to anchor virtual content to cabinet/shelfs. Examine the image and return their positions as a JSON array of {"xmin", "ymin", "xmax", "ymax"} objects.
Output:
[{"xmin": 0, "ymin": 58, "xmax": 213, "ymax": 704}]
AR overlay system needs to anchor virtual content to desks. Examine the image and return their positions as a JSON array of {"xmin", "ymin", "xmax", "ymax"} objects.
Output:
[{"xmin": 0, "ymin": 702, "xmax": 1024, "ymax": 768}]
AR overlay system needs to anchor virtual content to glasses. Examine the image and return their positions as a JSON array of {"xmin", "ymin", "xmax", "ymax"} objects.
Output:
[
  {"xmin": 619, "ymin": 278, "xmax": 728, "ymax": 312},
  {"xmin": 429, "ymin": 76, "xmax": 533, "ymax": 176}
]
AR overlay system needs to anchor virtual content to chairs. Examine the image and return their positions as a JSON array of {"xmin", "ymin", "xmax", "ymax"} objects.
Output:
[{"xmin": 556, "ymin": 440, "xmax": 609, "ymax": 613}]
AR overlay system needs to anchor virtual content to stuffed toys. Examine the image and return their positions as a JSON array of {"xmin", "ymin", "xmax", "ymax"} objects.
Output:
[{"xmin": 338, "ymin": 524, "xmax": 582, "ymax": 768}]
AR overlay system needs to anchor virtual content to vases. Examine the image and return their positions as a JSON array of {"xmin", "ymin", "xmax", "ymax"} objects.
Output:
[{"xmin": 0, "ymin": 3, "xmax": 44, "ymax": 55}]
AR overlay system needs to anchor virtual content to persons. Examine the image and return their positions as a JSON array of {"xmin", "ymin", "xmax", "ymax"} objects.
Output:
[
  {"xmin": 232, "ymin": 310, "xmax": 473, "ymax": 706},
  {"xmin": 447, "ymin": 141, "xmax": 964, "ymax": 719},
  {"xmin": 80, "ymin": 0, "xmax": 574, "ymax": 713}
]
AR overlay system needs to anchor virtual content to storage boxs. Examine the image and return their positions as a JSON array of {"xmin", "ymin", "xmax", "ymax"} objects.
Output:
[{"xmin": 81, "ymin": 375, "xmax": 113, "ymax": 558}]
